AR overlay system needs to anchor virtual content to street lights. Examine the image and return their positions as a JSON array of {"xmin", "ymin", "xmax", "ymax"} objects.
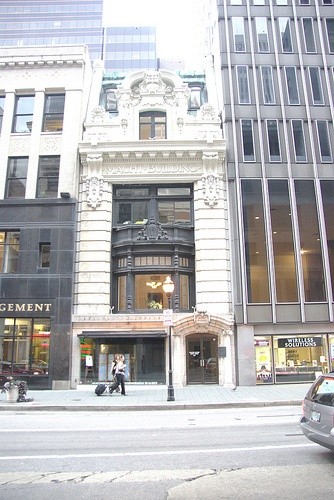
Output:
[{"xmin": 162, "ymin": 276, "xmax": 177, "ymax": 402}]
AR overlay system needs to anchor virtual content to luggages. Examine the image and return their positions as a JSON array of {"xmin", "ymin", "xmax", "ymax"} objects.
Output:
[{"xmin": 95, "ymin": 384, "xmax": 107, "ymax": 396}]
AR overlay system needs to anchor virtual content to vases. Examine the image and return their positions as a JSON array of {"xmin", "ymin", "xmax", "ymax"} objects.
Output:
[{"xmin": 6, "ymin": 385, "xmax": 19, "ymax": 402}]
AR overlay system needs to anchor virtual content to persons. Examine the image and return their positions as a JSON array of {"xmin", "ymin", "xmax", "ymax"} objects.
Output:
[
  {"xmin": 141, "ymin": 355, "xmax": 146, "ymax": 374},
  {"xmin": 109, "ymin": 354, "xmax": 127, "ymax": 396},
  {"xmin": 90, "ymin": 176, "xmax": 98, "ymax": 186},
  {"xmin": 207, "ymin": 174, "xmax": 215, "ymax": 184}
]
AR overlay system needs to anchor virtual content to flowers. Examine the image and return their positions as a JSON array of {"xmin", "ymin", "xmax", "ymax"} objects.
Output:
[{"xmin": 1, "ymin": 378, "xmax": 32, "ymax": 402}]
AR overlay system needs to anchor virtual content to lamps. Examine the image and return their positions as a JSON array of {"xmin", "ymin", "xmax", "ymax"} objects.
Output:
[{"xmin": 145, "ymin": 275, "xmax": 162, "ymax": 290}]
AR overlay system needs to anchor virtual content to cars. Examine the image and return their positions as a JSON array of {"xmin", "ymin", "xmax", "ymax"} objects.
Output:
[
  {"xmin": 205, "ymin": 358, "xmax": 217, "ymax": 375},
  {"xmin": 0, "ymin": 358, "xmax": 49, "ymax": 375},
  {"xmin": 299, "ymin": 371, "xmax": 334, "ymax": 453}
]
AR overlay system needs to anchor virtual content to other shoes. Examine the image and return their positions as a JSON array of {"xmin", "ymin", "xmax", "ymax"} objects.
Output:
[
  {"xmin": 116, "ymin": 391, "xmax": 120, "ymax": 393},
  {"xmin": 121, "ymin": 393, "xmax": 127, "ymax": 396},
  {"xmin": 109, "ymin": 392, "xmax": 112, "ymax": 396},
  {"xmin": 109, "ymin": 387, "xmax": 111, "ymax": 390}
]
